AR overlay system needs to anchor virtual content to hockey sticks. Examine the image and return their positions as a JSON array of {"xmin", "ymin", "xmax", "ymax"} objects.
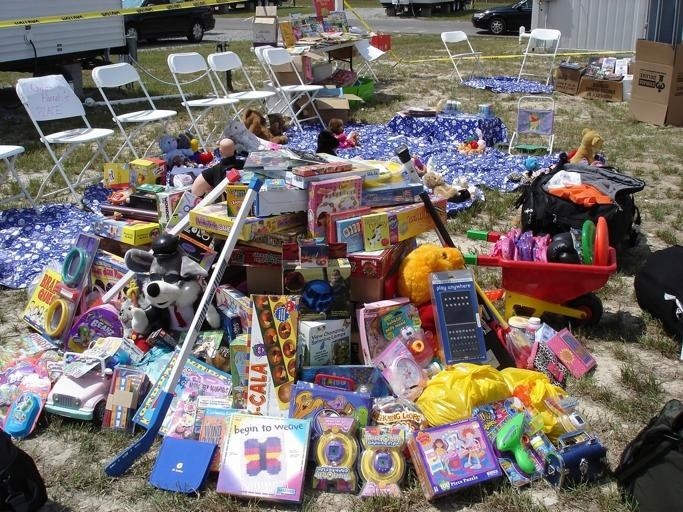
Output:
[
  {"xmin": 73, "ymin": 169, "xmax": 240, "ymax": 323},
  {"xmin": 106, "ymin": 178, "xmax": 262, "ymax": 477}
]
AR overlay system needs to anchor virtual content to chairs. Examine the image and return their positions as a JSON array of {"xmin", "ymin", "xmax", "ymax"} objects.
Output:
[
  {"xmin": 350, "ymin": 27, "xmax": 387, "ymax": 82},
  {"xmin": 517, "ymin": 28, "xmax": 561, "ymax": 86},
  {"xmin": 204, "ymin": 52, "xmax": 275, "ymax": 146},
  {"xmin": 441, "ymin": 30, "xmax": 483, "ymax": 83},
  {"xmin": 0, "ymin": 145, "xmax": 41, "ymax": 217},
  {"xmin": 92, "ymin": 63, "xmax": 177, "ymax": 163},
  {"xmin": 17, "ymin": 75, "xmax": 125, "ymax": 207},
  {"xmin": 255, "ymin": 45, "xmax": 327, "ymax": 134}
]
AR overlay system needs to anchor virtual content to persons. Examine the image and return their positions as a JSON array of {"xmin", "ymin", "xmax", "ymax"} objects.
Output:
[
  {"xmin": 329, "ymin": 118, "xmax": 357, "ymax": 148},
  {"xmin": 194, "ymin": 138, "xmax": 246, "ymax": 202}
]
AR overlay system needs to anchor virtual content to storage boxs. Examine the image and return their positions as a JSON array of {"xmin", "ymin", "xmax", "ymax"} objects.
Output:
[
  {"xmin": 309, "ymin": 87, "xmax": 362, "ymax": 126},
  {"xmin": 577, "ymin": 76, "xmax": 623, "ymax": 102},
  {"xmin": 168, "ymin": 52, "xmax": 239, "ymax": 154},
  {"xmin": 629, "ymin": 39, "xmax": 682, "ymax": 124},
  {"xmin": 555, "ymin": 67, "xmax": 585, "ymax": 95}
]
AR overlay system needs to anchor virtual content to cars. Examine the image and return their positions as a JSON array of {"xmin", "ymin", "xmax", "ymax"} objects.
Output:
[
  {"xmin": 115, "ymin": 0, "xmax": 214, "ymax": 46},
  {"xmin": 472, "ymin": 0, "xmax": 533, "ymax": 36}
]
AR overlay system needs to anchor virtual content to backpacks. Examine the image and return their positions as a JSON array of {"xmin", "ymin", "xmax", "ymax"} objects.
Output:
[
  {"xmin": 0, "ymin": 426, "xmax": 49, "ymax": 512},
  {"xmin": 521, "ymin": 154, "xmax": 645, "ymax": 255},
  {"xmin": 634, "ymin": 241, "xmax": 683, "ymax": 346},
  {"xmin": 615, "ymin": 399, "xmax": 683, "ymax": 512}
]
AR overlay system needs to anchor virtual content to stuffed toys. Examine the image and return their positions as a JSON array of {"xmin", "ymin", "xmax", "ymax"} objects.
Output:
[
  {"xmin": 159, "ymin": 133, "xmax": 194, "ymax": 165},
  {"xmin": 398, "ymin": 244, "xmax": 465, "ymax": 334},
  {"xmin": 2, "ymin": 98, "xmax": 615, "ymax": 498},
  {"xmin": 423, "ymin": 172, "xmax": 462, "ymax": 201},
  {"xmin": 244, "ymin": 109, "xmax": 288, "ymax": 145},
  {"xmin": 570, "ymin": 128, "xmax": 603, "ymax": 165},
  {"xmin": 125, "ymin": 233, "xmax": 224, "ymax": 336}
]
